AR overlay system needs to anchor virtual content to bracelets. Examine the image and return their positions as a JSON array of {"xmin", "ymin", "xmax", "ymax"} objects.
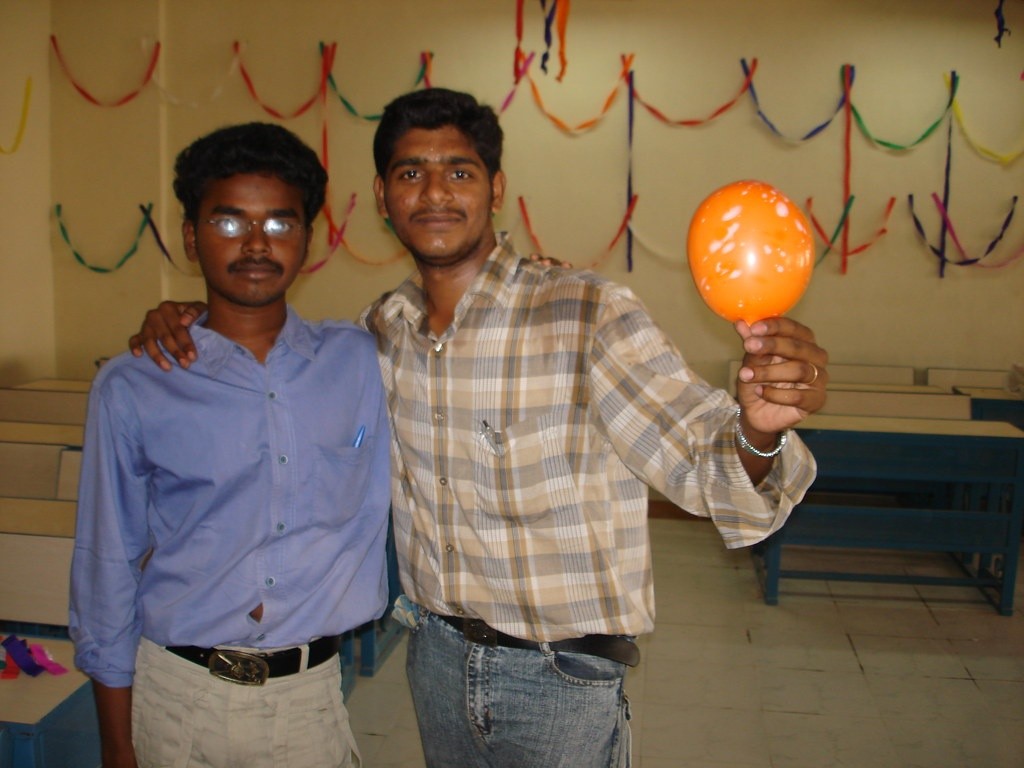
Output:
[{"xmin": 735, "ymin": 407, "xmax": 787, "ymax": 459}]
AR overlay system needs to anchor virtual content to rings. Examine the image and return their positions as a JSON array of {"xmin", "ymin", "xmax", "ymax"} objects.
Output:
[{"xmin": 804, "ymin": 361, "xmax": 818, "ymax": 385}]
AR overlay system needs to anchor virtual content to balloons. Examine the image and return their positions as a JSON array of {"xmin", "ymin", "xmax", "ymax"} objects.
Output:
[{"xmin": 688, "ymin": 179, "xmax": 816, "ymax": 330}]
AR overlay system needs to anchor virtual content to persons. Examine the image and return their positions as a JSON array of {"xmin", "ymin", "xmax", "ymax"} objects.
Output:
[
  {"xmin": 67, "ymin": 121, "xmax": 574, "ymax": 767},
  {"xmin": 127, "ymin": 87, "xmax": 831, "ymax": 768}
]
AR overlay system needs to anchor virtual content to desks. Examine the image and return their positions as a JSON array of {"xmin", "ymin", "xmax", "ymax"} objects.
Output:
[
  {"xmin": 0, "ymin": 630, "xmax": 104, "ymax": 768},
  {"xmin": 756, "ymin": 412, "xmax": 1024, "ymax": 617},
  {"xmin": 0, "ymin": 374, "xmax": 92, "ymax": 635},
  {"xmin": 953, "ymin": 384, "xmax": 1024, "ymax": 431}
]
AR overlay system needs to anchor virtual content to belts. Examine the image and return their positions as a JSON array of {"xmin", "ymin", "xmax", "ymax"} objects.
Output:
[
  {"xmin": 165, "ymin": 634, "xmax": 338, "ymax": 686},
  {"xmin": 438, "ymin": 614, "xmax": 640, "ymax": 667}
]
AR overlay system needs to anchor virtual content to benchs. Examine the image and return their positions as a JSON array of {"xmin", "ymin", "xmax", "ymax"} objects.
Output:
[
  {"xmin": 814, "ymin": 389, "xmax": 972, "ymax": 420},
  {"xmin": 726, "ymin": 360, "xmax": 915, "ymax": 401},
  {"xmin": 925, "ymin": 365, "xmax": 1012, "ymax": 395}
]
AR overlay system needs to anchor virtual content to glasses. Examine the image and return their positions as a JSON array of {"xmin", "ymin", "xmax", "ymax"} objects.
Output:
[{"xmin": 192, "ymin": 215, "xmax": 307, "ymax": 240}]
